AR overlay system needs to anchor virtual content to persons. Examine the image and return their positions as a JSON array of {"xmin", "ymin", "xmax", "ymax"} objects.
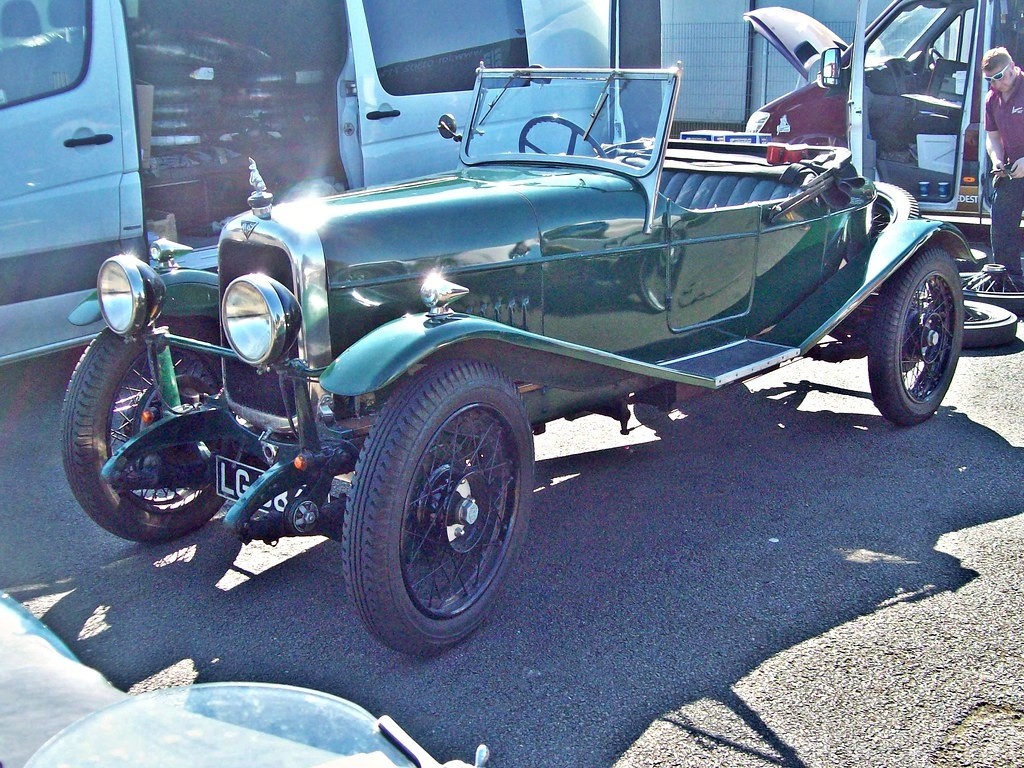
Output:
[{"xmin": 981, "ymin": 46, "xmax": 1024, "ymax": 276}]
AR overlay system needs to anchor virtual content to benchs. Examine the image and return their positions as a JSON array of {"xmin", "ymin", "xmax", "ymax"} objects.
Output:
[{"xmin": 658, "ymin": 172, "xmax": 799, "ymax": 209}]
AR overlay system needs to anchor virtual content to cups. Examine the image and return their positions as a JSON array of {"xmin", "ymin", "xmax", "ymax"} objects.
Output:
[
  {"xmin": 937, "ymin": 182, "xmax": 951, "ymax": 198},
  {"xmin": 766, "ymin": 142, "xmax": 809, "ymax": 165},
  {"xmin": 918, "ymin": 181, "xmax": 931, "ymax": 197}
]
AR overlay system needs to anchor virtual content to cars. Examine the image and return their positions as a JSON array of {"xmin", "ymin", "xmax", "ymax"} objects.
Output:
[{"xmin": 58, "ymin": 58, "xmax": 980, "ymax": 661}]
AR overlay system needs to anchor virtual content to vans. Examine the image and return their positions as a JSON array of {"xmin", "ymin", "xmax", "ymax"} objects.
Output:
[{"xmin": 1, "ymin": 0, "xmax": 631, "ymax": 379}]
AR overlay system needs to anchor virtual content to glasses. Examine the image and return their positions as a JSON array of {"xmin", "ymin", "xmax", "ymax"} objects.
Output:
[{"xmin": 985, "ymin": 62, "xmax": 1010, "ymax": 82}]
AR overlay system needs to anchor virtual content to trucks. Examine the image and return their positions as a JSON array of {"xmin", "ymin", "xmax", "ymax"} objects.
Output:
[{"xmin": 743, "ymin": 0, "xmax": 1024, "ymax": 238}]
